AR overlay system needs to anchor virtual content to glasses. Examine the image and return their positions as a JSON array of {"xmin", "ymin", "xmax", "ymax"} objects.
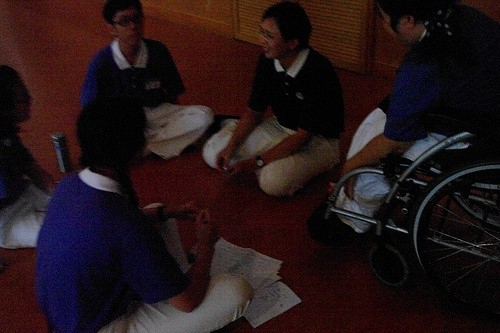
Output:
[{"xmin": 113, "ymin": 15, "xmax": 145, "ymax": 26}]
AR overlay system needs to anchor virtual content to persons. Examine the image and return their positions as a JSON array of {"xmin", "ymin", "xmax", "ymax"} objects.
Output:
[
  {"xmin": 78, "ymin": 0, "xmax": 212, "ymax": 161},
  {"xmin": 202, "ymin": 0, "xmax": 343, "ymax": 195},
  {"xmin": 0, "ymin": 64, "xmax": 54, "ymax": 250},
  {"xmin": 33, "ymin": 92, "xmax": 252, "ymax": 333},
  {"xmin": 321, "ymin": 1, "xmax": 500, "ymax": 233}
]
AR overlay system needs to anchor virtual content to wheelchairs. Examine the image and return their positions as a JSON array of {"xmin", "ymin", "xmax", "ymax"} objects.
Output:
[{"xmin": 307, "ymin": 96, "xmax": 500, "ymax": 297}]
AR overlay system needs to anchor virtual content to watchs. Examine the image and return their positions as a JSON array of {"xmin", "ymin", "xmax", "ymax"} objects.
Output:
[{"xmin": 256, "ymin": 155, "xmax": 265, "ymax": 169}]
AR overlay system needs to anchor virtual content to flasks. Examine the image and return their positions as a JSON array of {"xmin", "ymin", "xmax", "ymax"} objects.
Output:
[{"xmin": 52, "ymin": 133, "xmax": 72, "ymax": 172}]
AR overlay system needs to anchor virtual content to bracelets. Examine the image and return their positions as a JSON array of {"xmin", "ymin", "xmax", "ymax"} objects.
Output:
[{"xmin": 157, "ymin": 206, "xmax": 166, "ymax": 222}]
